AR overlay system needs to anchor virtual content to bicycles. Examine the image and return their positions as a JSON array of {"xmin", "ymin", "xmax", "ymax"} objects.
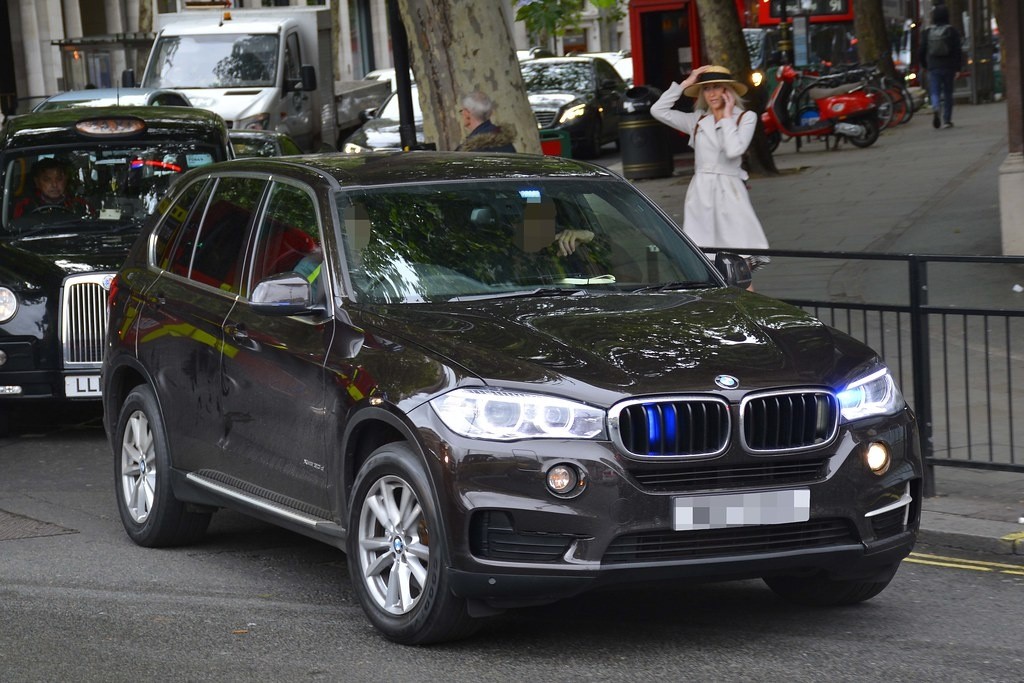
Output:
[{"xmin": 781, "ymin": 62, "xmax": 913, "ymax": 145}]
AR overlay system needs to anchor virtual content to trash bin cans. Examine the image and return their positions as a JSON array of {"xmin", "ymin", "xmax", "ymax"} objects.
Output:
[{"xmin": 615, "ymin": 84, "xmax": 677, "ymax": 177}]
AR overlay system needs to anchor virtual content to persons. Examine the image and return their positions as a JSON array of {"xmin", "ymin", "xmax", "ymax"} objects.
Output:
[
  {"xmin": 918, "ymin": 6, "xmax": 962, "ymax": 129},
  {"xmin": 454, "ymin": 92, "xmax": 517, "ymax": 154},
  {"xmin": 294, "ymin": 198, "xmax": 404, "ymax": 309},
  {"xmin": 440, "ymin": 189, "xmax": 643, "ymax": 293},
  {"xmin": 650, "ymin": 65, "xmax": 771, "ymax": 294},
  {"xmin": 9, "ymin": 157, "xmax": 87, "ymax": 226}
]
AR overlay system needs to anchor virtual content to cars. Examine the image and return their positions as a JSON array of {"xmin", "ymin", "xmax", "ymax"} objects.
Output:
[
  {"xmin": 363, "ymin": 45, "xmax": 634, "ymax": 88},
  {"xmin": 226, "ymin": 132, "xmax": 300, "ymax": 161},
  {"xmin": 0, "ymin": 107, "xmax": 237, "ymax": 424},
  {"xmin": 30, "ymin": 88, "xmax": 212, "ymax": 163},
  {"xmin": 519, "ymin": 58, "xmax": 627, "ymax": 156},
  {"xmin": 343, "ymin": 81, "xmax": 439, "ymax": 150}
]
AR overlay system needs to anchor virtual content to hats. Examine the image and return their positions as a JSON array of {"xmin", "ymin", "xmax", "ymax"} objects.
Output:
[{"xmin": 684, "ymin": 65, "xmax": 748, "ymax": 98}]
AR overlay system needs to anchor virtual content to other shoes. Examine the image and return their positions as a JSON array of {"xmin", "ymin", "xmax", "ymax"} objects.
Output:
[
  {"xmin": 933, "ymin": 110, "xmax": 941, "ymax": 129},
  {"xmin": 944, "ymin": 122, "xmax": 953, "ymax": 128}
]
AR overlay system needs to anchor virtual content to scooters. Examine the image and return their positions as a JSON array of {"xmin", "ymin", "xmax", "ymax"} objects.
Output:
[{"xmin": 762, "ymin": 61, "xmax": 879, "ymax": 155}]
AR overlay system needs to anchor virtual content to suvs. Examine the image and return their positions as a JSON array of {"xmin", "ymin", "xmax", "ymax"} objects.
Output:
[{"xmin": 98, "ymin": 151, "xmax": 923, "ymax": 646}]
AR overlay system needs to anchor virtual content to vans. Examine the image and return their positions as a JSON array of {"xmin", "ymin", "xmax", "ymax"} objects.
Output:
[{"xmin": 744, "ymin": 27, "xmax": 787, "ymax": 92}]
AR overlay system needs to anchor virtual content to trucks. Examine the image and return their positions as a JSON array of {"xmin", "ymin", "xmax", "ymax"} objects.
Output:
[{"xmin": 122, "ymin": 12, "xmax": 392, "ymax": 147}]
